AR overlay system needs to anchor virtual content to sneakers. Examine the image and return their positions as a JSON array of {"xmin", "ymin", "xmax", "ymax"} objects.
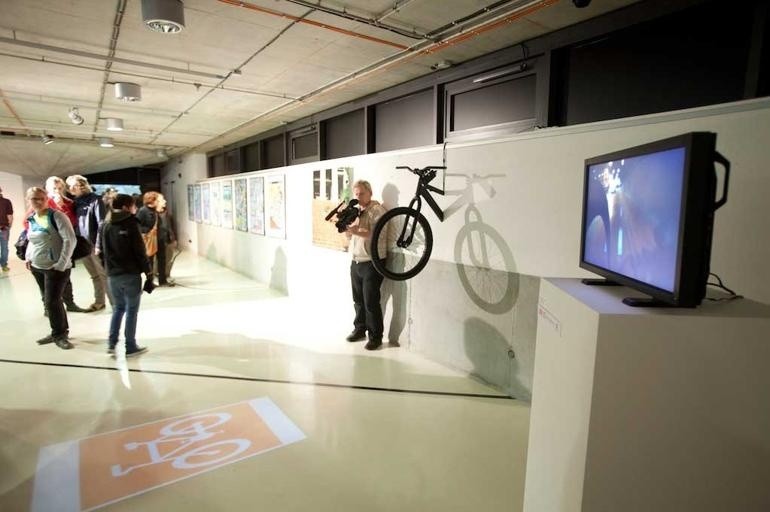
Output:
[
  {"xmin": 37, "ymin": 336, "xmax": 54, "ymax": 344},
  {"xmin": 347, "ymin": 329, "xmax": 365, "ymax": 341},
  {"xmin": 56, "ymin": 338, "xmax": 73, "ymax": 349},
  {"xmin": 68, "ymin": 303, "xmax": 84, "ymax": 311},
  {"xmin": 126, "ymin": 345, "xmax": 146, "ymax": 357},
  {"xmin": 366, "ymin": 337, "xmax": 382, "ymax": 349},
  {"xmin": 91, "ymin": 303, "xmax": 105, "ymax": 310},
  {"xmin": 107, "ymin": 344, "xmax": 114, "ymax": 353}
]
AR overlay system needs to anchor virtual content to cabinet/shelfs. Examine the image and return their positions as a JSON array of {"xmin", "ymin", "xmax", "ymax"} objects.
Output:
[{"xmin": 522, "ymin": 276, "xmax": 770, "ymax": 512}]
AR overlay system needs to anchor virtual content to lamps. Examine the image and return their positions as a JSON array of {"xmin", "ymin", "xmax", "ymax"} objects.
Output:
[{"xmin": 39, "ymin": 0, "xmax": 185, "ymax": 148}]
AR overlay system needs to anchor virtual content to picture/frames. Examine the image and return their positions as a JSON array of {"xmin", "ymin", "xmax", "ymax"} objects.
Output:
[{"xmin": 187, "ymin": 176, "xmax": 266, "ymax": 236}]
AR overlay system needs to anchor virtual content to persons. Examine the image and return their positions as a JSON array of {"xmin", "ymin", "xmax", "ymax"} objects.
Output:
[
  {"xmin": 0, "ymin": 186, "xmax": 15, "ymax": 273},
  {"xmin": 90, "ymin": 184, "xmax": 176, "ymax": 293},
  {"xmin": 93, "ymin": 194, "xmax": 152, "ymax": 359},
  {"xmin": 65, "ymin": 173, "xmax": 115, "ymax": 312},
  {"xmin": 23, "ymin": 186, "xmax": 77, "ymax": 350},
  {"xmin": 24, "ymin": 176, "xmax": 89, "ymax": 317},
  {"xmin": 343, "ymin": 180, "xmax": 387, "ymax": 351}
]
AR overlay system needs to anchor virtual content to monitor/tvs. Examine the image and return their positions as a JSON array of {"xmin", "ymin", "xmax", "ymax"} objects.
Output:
[{"xmin": 578, "ymin": 130, "xmax": 732, "ymax": 309}]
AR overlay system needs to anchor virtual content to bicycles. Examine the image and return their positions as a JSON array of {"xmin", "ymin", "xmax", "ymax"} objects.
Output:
[{"xmin": 373, "ymin": 166, "xmax": 444, "ymax": 280}]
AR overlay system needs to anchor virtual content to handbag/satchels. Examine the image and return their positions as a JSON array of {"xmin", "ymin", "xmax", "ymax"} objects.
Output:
[
  {"xmin": 142, "ymin": 225, "xmax": 158, "ymax": 256},
  {"xmin": 15, "ymin": 238, "xmax": 27, "ymax": 260},
  {"xmin": 72, "ymin": 236, "xmax": 90, "ymax": 259}
]
